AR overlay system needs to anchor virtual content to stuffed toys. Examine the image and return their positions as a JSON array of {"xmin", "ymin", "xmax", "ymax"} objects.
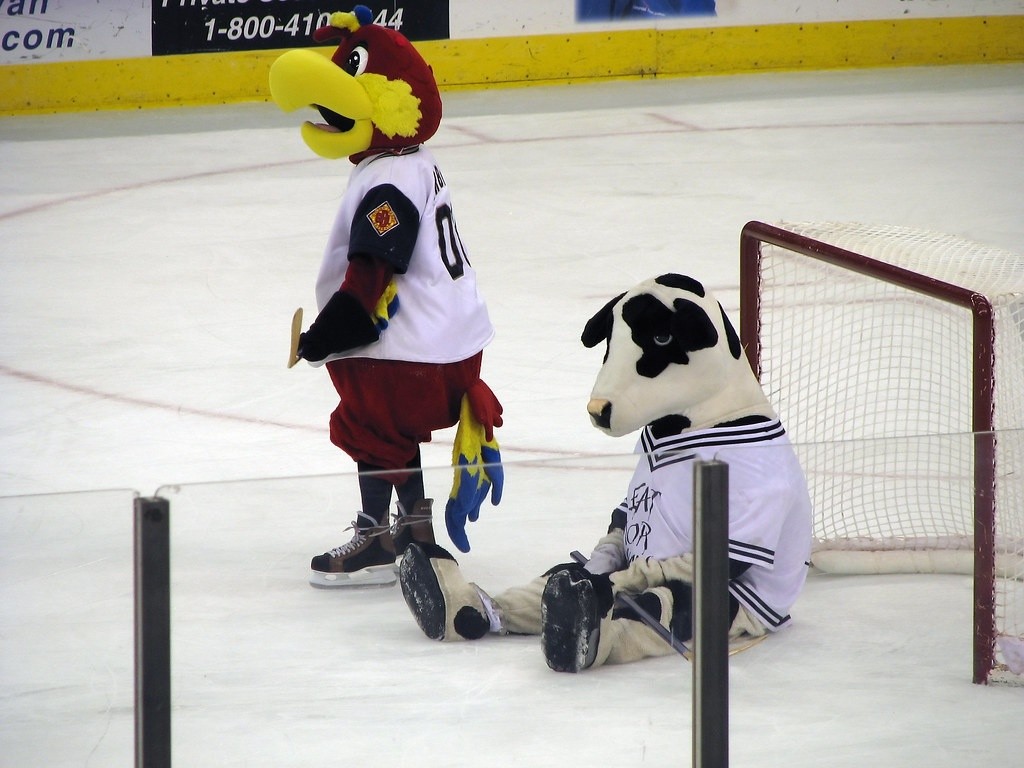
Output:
[
  {"xmin": 269, "ymin": 8, "xmax": 502, "ymax": 587},
  {"xmin": 398, "ymin": 274, "xmax": 813, "ymax": 673}
]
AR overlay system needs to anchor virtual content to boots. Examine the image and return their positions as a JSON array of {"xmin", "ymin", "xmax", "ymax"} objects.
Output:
[
  {"xmin": 389, "ymin": 498, "xmax": 436, "ymax": 567},
  {"xmin": 308, "ymin": 506, "xmax": 399, "ymax": 586}
]
